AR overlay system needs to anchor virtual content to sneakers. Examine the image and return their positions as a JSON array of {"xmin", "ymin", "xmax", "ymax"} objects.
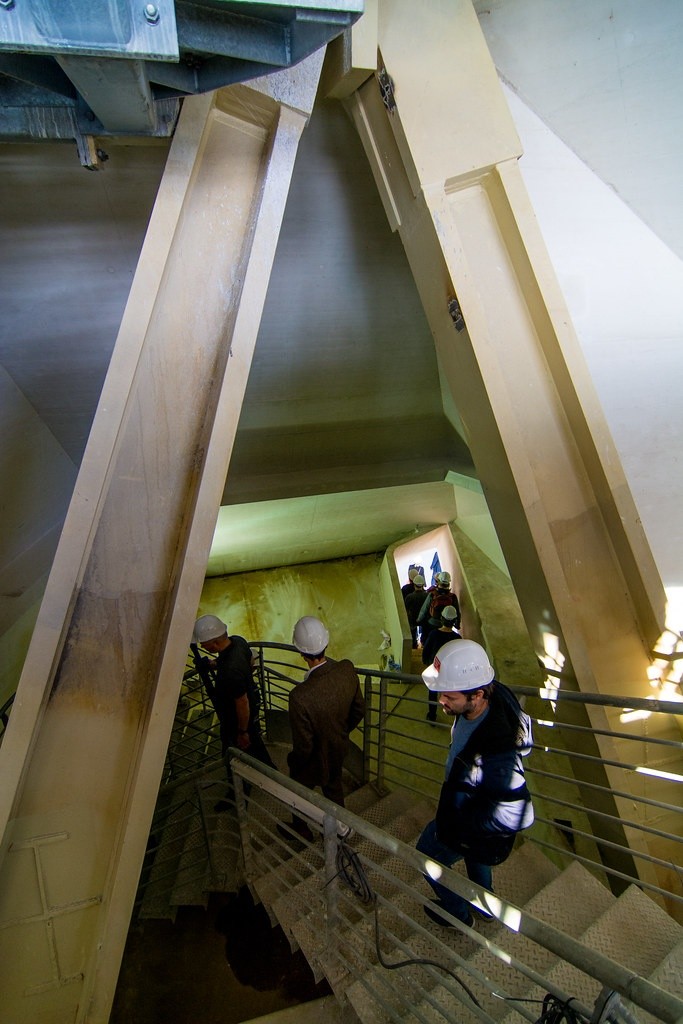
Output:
[
  {"xmin": 423, "ymin": 898, "xmax": 476, "ymax": 931},
  {"xmin": 476, "ymin": 908, "xmax": 495, "ymax": 922}
]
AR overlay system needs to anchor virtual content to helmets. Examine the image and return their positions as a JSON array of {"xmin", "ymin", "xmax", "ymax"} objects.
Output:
[
  {"xmin": 441, "ymin": 605, "xmax": 457, "ymax": 620},
  {"xmin": 408, "ymin": 569, "xmax": 418, "ymax": 581},
  {"xmin": 190, "ymin": 614, "xmax": 228, "ymax": 643},
  {"xmin": 437, "ymin": 571, "xmax": 452, "ymax": 585},
  {"xmin": 413, "ymin": 575, "xmax": 425, "ymax": 586},
  {"xmin": 421, "ymin": 639, "xmax": 495, "ymax": 690},
  {"xmin": 292, "ymin": 615, "xmax": 330, "ymax": 654}
]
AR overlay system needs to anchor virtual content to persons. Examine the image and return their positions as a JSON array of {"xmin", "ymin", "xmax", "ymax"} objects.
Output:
[
  {"xmin": 401, "ymin": 569, "xmax": 462, "ymax": 727},
  {"xmin": 416, "ymin": 639, "xmax": 534, "ymax": 934},
  {"xmin": 191, "ymin": 614, "xmax": 279, "ymax": 812},
  {"xmin": 274, "ymin": 615, "xmax": 362, "ymax": 841}
]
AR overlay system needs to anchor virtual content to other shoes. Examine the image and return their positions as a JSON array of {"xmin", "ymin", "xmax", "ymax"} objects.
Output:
[{"xmin": 276, "ymin": 821, "xmax": 309, "ymax": 839}]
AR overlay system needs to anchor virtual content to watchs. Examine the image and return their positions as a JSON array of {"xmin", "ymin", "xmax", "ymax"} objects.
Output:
[{"xmin": 237, "ymin": 729, "xmax": 247, "ymax": 735}]
